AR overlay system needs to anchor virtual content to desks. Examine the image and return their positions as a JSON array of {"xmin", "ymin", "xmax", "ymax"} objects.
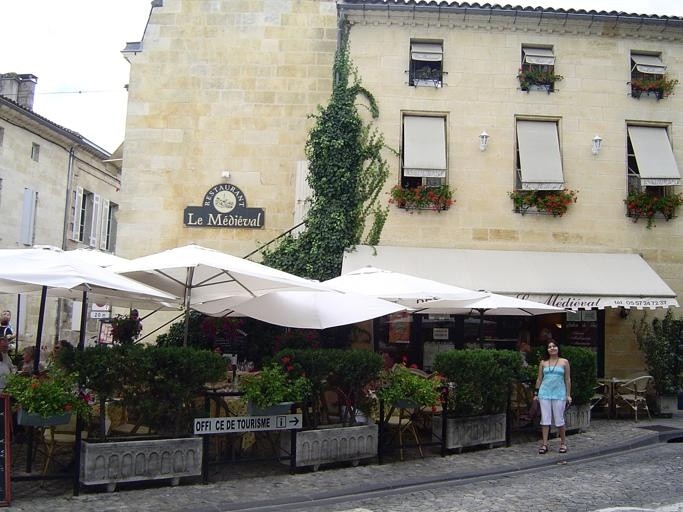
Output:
[{"xmin": 593, "ymin": 374, "xmax": 634, "ymax": 416}]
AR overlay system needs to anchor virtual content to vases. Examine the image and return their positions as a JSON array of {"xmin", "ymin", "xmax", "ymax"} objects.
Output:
[
  {"xmin": 387, "ymin": 397, "xmax": 422, "ymax": 409},
  {"xmin": 16, "ymin": 408, "xmax": 71, "ymax": 427},
  {"xmin": 247, "ymin": 401, "xmax": 293, "ymax": 416}
]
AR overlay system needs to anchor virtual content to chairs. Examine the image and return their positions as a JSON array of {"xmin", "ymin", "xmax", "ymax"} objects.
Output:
[
  {"xmin": 615, "ymin": 376, "xmax": 654, "ymax": 421},
  {"xmin": 585, "ymin": 378, "xmax": 611, "ymax": 417}
]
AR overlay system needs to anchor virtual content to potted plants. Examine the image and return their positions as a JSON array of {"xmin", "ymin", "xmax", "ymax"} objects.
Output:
[
  {"xmin": 631, "ymin": 308, "xmax": 683, "ymax": 415},
  {"xmin": 434, "ymin": 349, "xmax": 521, "ymax": 450},
  {"xmin": 529, "ymin": 342, "xmax": 597, "ymax": 435},
  {"xmin": 283, "ymin": 345, "xmax": 380, "ymax": 473},
  {"xmin": 57, "ymin": 336, "xmax": 228, "ymax": 493},
  {"xmin": 379, "ymin": 371, "xmax": 441, "ymax": 410},
  {"xmin": 238, "ymin": 357, "xmax": 312, "ymax": 417}
]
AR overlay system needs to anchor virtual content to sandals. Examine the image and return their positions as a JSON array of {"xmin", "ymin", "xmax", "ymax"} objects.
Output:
[
  {"xmin": 559, "ymin": 442, "xmax": 568, "ymax": 453},
  {"xmin": 539, "ymin": 445, "xmax": 549, "ymax": 454}
]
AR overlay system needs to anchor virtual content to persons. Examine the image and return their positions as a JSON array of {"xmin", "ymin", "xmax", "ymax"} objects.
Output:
[
  {"xmin": 532, "ymin": 338, "xmax": 573, "ymax": 454},
  {"xmin": 0, "ymin": 336, "xmax": 16, "ymax": 382}
]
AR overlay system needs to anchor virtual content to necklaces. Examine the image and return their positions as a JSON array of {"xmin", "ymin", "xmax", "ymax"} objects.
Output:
[{"xmin": 549, "ymin": 356, "xmax": 558, "ymax": 373}]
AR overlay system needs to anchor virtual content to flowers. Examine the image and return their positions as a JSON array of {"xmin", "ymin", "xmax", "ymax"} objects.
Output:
[{"xmin": 2, "ymin": 374, "xmax": 99, "ymax": 425}]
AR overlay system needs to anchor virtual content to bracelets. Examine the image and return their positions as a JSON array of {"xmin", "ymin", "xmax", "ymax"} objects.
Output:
[
  {"xmin": 533, "ymin": 394, "xmax": 539, "ymax": 395},
  {"xmin": 534, "ymin": 387, "xmax": 538, "ymax": 392}
]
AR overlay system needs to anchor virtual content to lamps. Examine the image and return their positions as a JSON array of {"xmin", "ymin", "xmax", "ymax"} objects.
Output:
[
  {"xmin": 477, "ymin": 131, "xmax": 489, "ymax": 151},
  {"xmin": 589, "ymin": 134, "xmax": 602, "ymax": 156}
]
